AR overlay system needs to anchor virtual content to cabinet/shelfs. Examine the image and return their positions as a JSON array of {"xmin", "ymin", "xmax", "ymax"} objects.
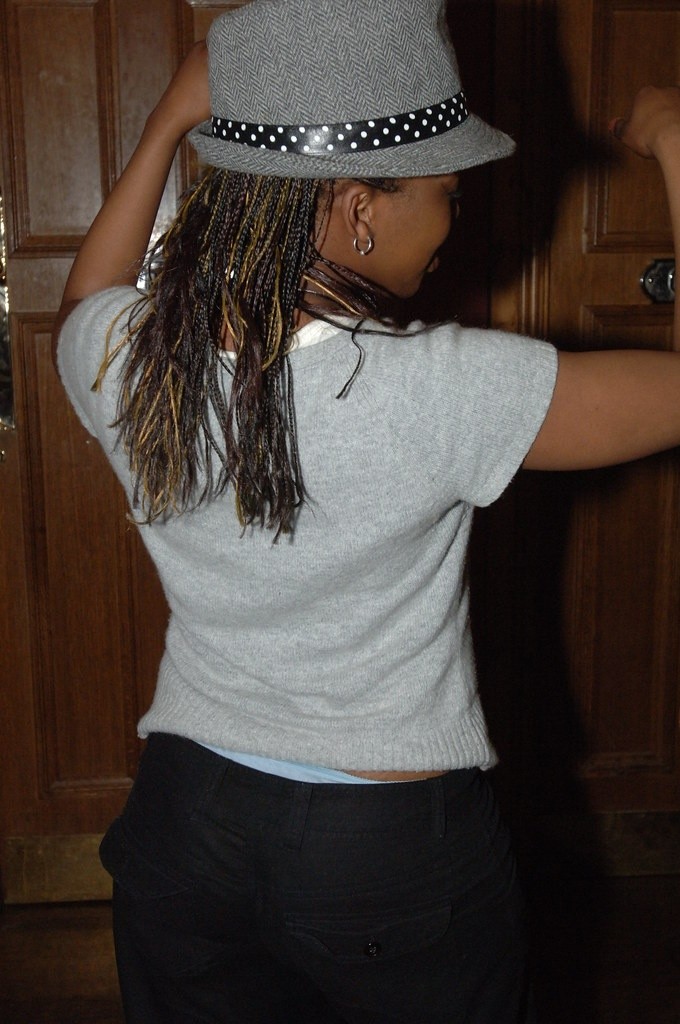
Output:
[{"xmin": 0, "ymin": 0, "xmax": 680, "ymax": 905}]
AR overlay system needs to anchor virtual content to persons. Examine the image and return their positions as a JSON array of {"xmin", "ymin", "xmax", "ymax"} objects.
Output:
[{"xmin": 52, "ymin": 2, "xmax": 679, "ymax": 1024}]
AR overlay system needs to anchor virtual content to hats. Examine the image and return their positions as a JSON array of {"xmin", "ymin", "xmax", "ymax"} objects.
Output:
[{"xmin": 186, "ymin": 0, "xmax": 515, "ymax": 179}]
